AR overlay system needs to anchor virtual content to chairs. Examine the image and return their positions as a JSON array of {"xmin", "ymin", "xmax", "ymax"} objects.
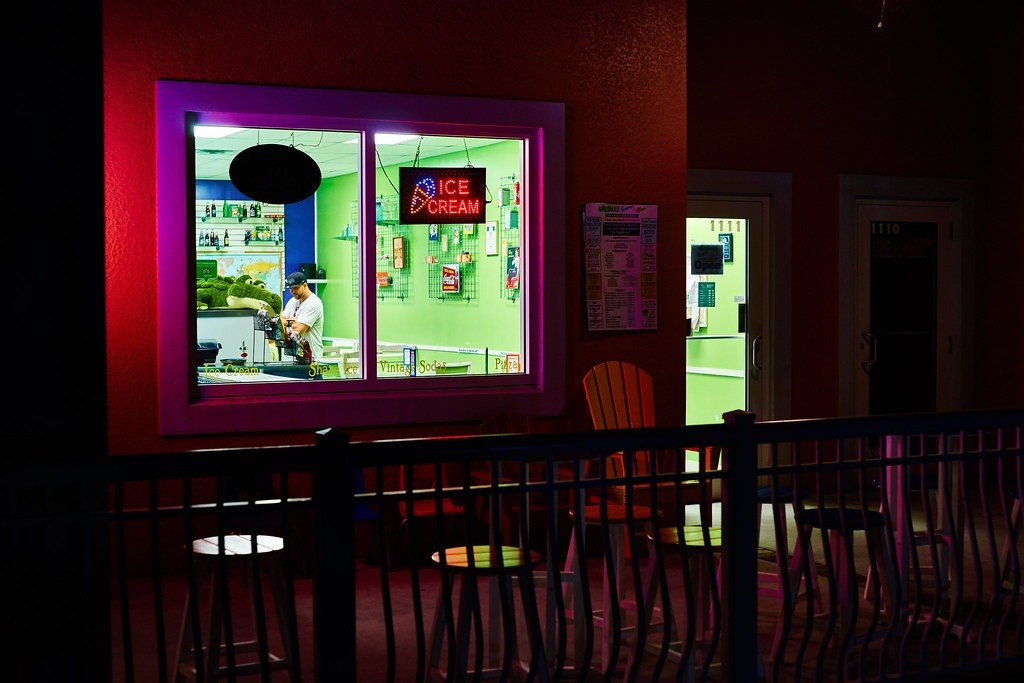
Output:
[
  {"xmin": 583, "ymin": 361, "xmax": 702, "ymax": 599},
  {"xmin": 321, "ymin": 343, "xmax": 383, "ymax": 376},
  {"xmin": 432, "ymin": 362, "xmax": 472, "ymax": 374},
  {"xmin": 389, "ymin": 461, "xmax": 465, "ymax": 555},
  {"xmin": 464, "ymin": 409, "xmax": 578, "ymax": 555}
]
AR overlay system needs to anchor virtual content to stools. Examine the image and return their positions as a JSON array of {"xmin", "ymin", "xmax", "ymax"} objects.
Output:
[
  {"xmin": 990, "ymin": 489, "xmax": 1024, "ymax": 595},
  {"xmin": 771, "ymin": 509, "xmax": 897, "ymax": 669},
  {"xmin": 562, "ymin": 504, "xmax": 682, "ymax": 678},
  {"xmin": 426, "ymin": 545, "xmax": 540, "ymax": 683},
  {"xmin": 626, "ymin": 528, "xmax": 727, "ymax": 683},
  {"xmin": 171, "ymin": 534, "xmax": 298, "ymax": 683}
]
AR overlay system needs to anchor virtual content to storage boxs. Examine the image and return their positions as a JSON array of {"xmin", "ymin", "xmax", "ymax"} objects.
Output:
[{"xmin": 403, "ymin": 347, "xmax": 416, "ymax": 376}]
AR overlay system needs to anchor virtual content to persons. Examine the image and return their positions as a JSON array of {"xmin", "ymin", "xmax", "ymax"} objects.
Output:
[{"xmin": 280, "ymin": 272, "xmax": 324, "ymax": 362}]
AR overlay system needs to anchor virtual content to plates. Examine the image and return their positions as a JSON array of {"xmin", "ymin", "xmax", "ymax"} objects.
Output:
[{"xmin": 220, "ymin": 359, "xmax": 247, "ymax": 365}]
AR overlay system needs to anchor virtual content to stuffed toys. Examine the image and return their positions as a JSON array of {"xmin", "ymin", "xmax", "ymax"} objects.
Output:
[{"xmin": 196, "ymin": 275, "xmax": 282, "ymax": 318}]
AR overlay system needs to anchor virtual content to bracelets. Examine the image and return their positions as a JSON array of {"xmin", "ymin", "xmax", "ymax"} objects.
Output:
[{"xmin": 286, "ymin": 320, "xmax": 295, "ymax": 327}]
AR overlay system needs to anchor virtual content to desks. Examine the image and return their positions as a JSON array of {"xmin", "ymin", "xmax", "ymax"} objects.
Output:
[
  {"xmin": 198, "ymin": 310, "xmax": 265, "ymax": 366},
  {"xmin": 316, "ymin": 353, "xmax": 403, "ymax": 365}
]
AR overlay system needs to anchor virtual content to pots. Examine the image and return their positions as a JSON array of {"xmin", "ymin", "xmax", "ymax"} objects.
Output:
[{"xmin": 197, "ymin": 339, "xmax": 222, "ymax": 365}]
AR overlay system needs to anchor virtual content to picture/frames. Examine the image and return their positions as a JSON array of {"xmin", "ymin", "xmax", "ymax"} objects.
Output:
[{"xmin": 393, "ymin": 236, "xmax": 404, "ymax": 269}]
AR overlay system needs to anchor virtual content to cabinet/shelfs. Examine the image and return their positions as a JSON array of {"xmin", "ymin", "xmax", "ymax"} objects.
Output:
[{"xmin": 196, "ymin": 216, "xmax": 285, "ymax": 253}]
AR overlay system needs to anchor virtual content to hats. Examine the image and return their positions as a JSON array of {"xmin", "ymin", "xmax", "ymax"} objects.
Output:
[{"xmin": 285, "ymin": 272, "xmax": 307, "ymax": 289}]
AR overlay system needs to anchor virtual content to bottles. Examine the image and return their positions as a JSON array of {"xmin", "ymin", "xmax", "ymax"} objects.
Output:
[
  {"xmin": 199, "ymin": 231, "xmax": 204, "ymax": 246},
  {"xmin": 223, "ymin": 205, "xmax": 228, "ymax": 217},
  {"xmin": 244, "ymin": 229, "xmax": 251, "ymax": 244},
  {"xmin": 256, "ymin": 203, "xmax": 261, "ymax": 218},
  {"xmin": 250, "ymin": 203, "xmax": 256, "ymax": 217},
  {"xmin": 210, "ymin": 232, "xmax": 214, "ymax": 245},
  {"xmin": 214, "ymin": 236, "xmax": 219, "ymax": 246},
  {"xmin": 238, "ymin": 204, "xmax": 241, "ymax": 216},
  {"xmin": 239, "ymin": 341, "xmax": 249, "ymax": 363},
  {"xmin": 224, "ymin": 229, "xmax": 230, "ymax": 246},
  {"xmin": 212, "ymin": 203, "xmax": 216, "ymax": 217},
  {"xmin": 279, "ymin": 227, "xmax": 283, "ymax": 241},
  {"xmin": 270, "ymin": 229, "xmax": 275, "ymax": 238},
  {"xmin": 205, "ymin": 233, "xmax": 209, "ymax": 246},
  {"xmin": 243, "ymin": 204, "xmax": 247, "ymax": 217},
  {"xmin": 206, "ymin": 205, "xmax": 210, "ymax": 217},
  {"xmin": 255, "ymin": 205, "xmax": 257, "ymax": 208},
  {"xmin": 223, "ymin": 200, "xmax": 228, "ymax": 210}
]
